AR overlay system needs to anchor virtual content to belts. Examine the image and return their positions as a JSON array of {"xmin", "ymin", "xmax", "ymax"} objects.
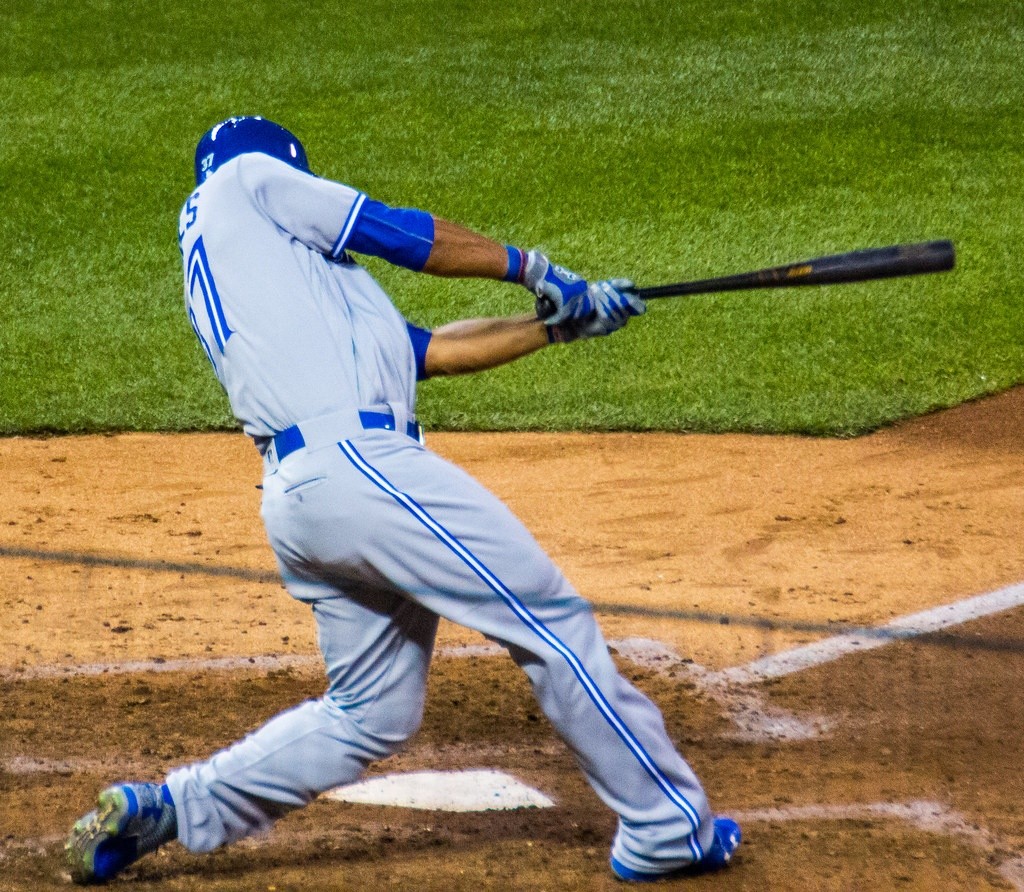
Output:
[{"xmin": 274, "ymin": 411, "xmax": 426, "ymax": 462}]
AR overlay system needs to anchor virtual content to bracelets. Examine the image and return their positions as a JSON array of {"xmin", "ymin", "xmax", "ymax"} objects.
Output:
[{"xmin": 505, "ymin": 245, "xmax": 520, "ymax": 281}]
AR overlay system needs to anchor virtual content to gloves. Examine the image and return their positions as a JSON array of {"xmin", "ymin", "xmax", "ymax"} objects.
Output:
[
  {"xmin": 524, "ymin": 250, "xmax": 594, "ymax": 329},
  {"xmin": 560, "ymin": 278, "xmax": 647, "ymax": 343}
]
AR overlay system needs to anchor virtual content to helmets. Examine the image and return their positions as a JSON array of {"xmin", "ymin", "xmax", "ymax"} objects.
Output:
[{"xmin": 195, "ymin": 117, "xmax": 316, "ymax": 190}]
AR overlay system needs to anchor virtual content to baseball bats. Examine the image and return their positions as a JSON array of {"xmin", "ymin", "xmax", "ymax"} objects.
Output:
[{"xmin": 537, "ymin": 241, "xmax": 955, "ymax": 319}]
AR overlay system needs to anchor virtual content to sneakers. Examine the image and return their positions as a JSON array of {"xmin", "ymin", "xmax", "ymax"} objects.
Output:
[
  {"xmin": 610, "ymin": 817, "xmax": 741, "ymax": 883},
  {"xmin": 64, "ymin": 782, "xmax": 177, "ymax": 887}
]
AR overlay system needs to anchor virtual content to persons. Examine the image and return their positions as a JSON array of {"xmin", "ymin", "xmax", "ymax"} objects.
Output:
[{"xmin": 66, "ymin": 117, "xmax": 740, "ymax": 885}]
